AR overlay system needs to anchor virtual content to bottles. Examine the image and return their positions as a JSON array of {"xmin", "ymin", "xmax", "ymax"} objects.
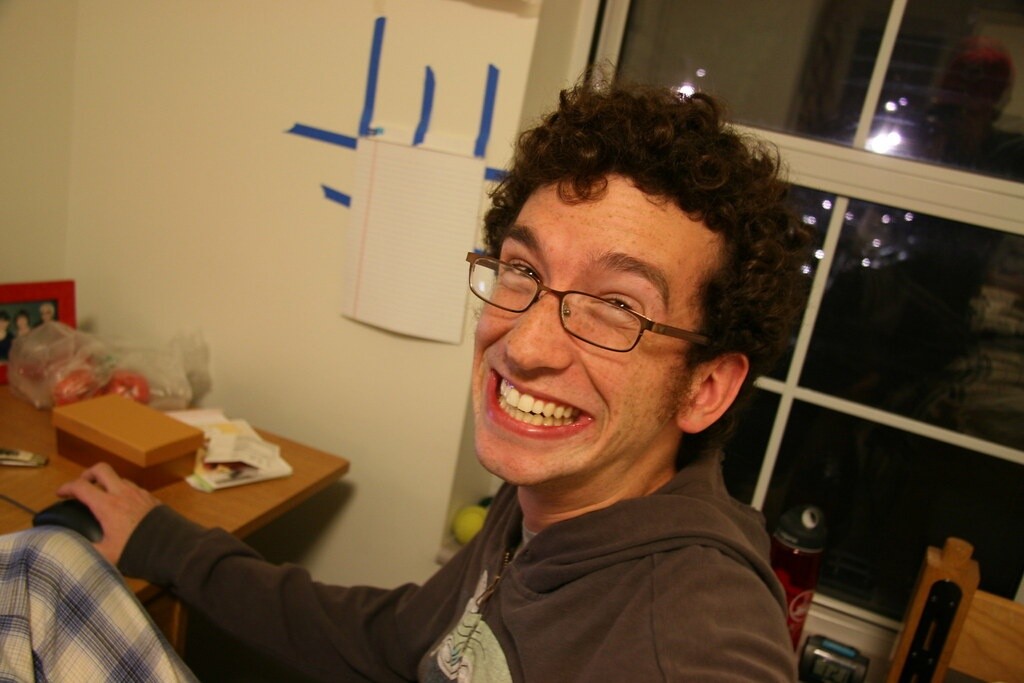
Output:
[{"xmin": 768, "ymin": 503, "xmax": 827, "ymax": 654}]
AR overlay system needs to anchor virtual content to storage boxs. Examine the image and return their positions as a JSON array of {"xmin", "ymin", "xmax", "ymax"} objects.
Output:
[{"xmin": 52, "ymin": 394, "xmax": 205, "ymax": 493}]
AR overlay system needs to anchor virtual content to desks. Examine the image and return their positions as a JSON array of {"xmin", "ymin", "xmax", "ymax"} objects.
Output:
[{"xmin": 0, "ymin": 358, "xmax": 353, "ymax": 658}]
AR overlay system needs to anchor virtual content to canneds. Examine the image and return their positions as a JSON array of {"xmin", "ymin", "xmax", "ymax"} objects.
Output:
[{"xmin": 798, "ymin": 632, "xmax": 871, "ymax": 683}]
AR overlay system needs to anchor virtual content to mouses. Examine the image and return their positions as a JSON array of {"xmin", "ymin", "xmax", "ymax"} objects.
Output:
[{"xmin": 31, "ymin": 497, "xmax": 106, "ymax": 545}]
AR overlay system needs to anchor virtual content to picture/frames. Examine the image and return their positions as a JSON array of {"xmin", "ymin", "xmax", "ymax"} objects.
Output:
[{"xmin": 0, "ymin": 278, "xmax": 77, "ymax": 384}]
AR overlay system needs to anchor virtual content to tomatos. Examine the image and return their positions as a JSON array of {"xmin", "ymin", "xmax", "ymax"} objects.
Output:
[{"xmin": 48, "ymin": 364, "xmax": 149, "ymax": 406}]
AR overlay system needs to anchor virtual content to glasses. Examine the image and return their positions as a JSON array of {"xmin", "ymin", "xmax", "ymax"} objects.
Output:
[{"xmin": 466, "ymin": 250, "xmax": 712, "ymax": 353}]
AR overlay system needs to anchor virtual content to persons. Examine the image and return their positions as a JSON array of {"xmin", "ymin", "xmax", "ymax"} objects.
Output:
[
  {"xmin": 0, "ymin": 80, "xmax": 812, "ymax": 683},
  {"xmin": 34, "ymin": 302, "xmax": 56, "ymax": 329},
  {"xmin": 0, "ymin": 310, "xmax": 16, "ymax": 362},
  {"xmin": 9, "ymin": 307, "xmax": 33, "ymax": 357}
]
